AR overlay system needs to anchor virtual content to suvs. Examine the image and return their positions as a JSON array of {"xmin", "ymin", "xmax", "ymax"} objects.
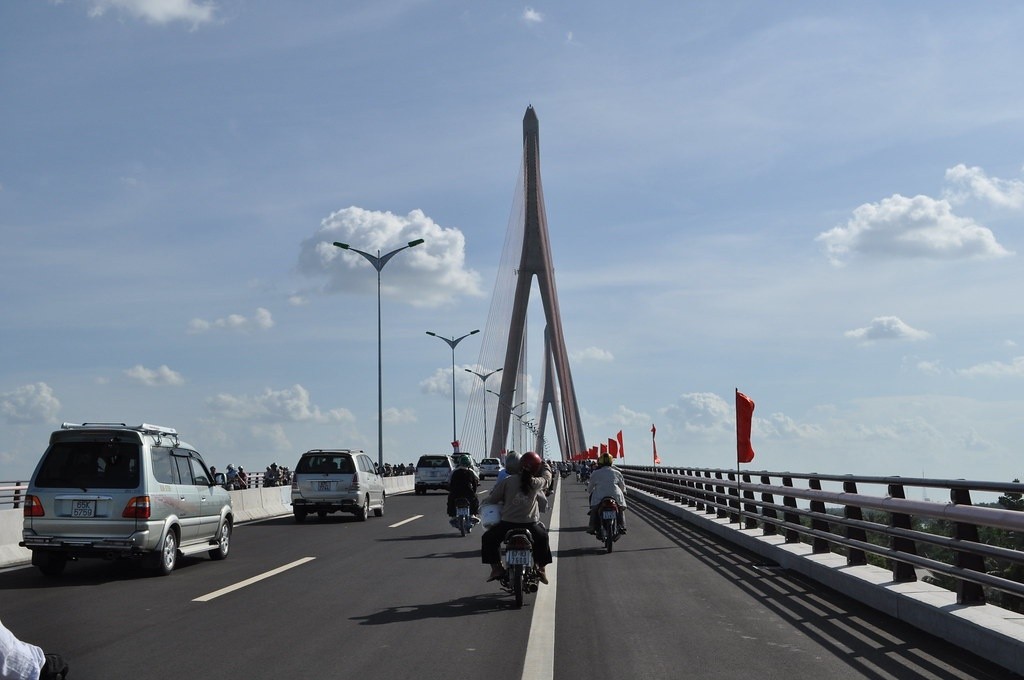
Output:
[
  {"xmin": 479, "ymin": 458, "xmax": 505, "ymax": 480},
  {"xmin": 20, "ymin": 422, "xmax": 234, "ymax": 577},
  {"xmin": 414, "ymin": 454, "xmax": 455, "ymax": 496},
  {"xmin": 290, "ymin": 448, "xmax": 387, "ymax": 522}
]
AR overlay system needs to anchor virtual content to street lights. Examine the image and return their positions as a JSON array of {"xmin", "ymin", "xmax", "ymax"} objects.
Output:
[
  {"xmin": 487, "ymin": 389, "xmax": 539, "ymax": 455},
  {"xmin": 426, "ymin": 329, "xmax": 480, "ymax": 440},
  {"xmin": 464, "ymin": 368, "xmax": 503, "ymax": 457},
  {"xmin": 333, "ymin": 238, "xmax": 424, "ymax": 463}
]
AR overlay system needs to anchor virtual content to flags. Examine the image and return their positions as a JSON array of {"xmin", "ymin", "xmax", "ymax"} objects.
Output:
[
  {"xmin": 651, "ymin": 425, "xmax": 660, "ymax": 464},
  {"xmin": 736, "ymin": 391, "xmax": 755, "ymax": 463},
  {"xmin": 616, "ymin": 431, "xmax": 624, "ymax": 457},
  {"xmin": 608, "ymin": 438, "xmax": 618, "ymax": 458},
  {"xmin": 601, "ymin": 443, "xmax": 607, "ymax": 455},
  {"xmin": 592, "ymin": 446, "xmax": 598, "ymax": 459},
  {"xmin": 589, "ymin": 448, "xmax": 593, "ymax": 459}
]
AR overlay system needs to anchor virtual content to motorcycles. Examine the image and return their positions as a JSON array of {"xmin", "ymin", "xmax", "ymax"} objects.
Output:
[
  {"xmin": 442, "ymin": 481, "xmax": 480, "ymax": 536},
  {"xmin": 584, "ymin": 488, "xmax": 627, "ymax": 553},
  {"xmin": 487, "ymin": 488, "xmax": 554, "ymax": 608},
  {"xmin": 543, "ymin": 469, "xmax": 591, "ymax": 498}
]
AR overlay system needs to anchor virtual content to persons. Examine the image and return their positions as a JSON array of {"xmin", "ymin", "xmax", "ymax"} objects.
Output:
[
  {"xmin": 447, "ymin": 451, "xmax": 627, "ymax": 584},
  {"xmin": 374, "ymin": 461, "xmax": 416, "ymax": 477},
  {"xmin": 210, "ymin": 462, "xmax": 292, "ymax": 490},
  {"xmin": 0, "ymin": 620, "xmax": 68, "ymax": 680}
]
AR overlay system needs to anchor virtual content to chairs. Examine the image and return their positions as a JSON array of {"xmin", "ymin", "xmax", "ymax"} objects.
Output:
[{"xmin": 321, "ymin": 461, "xmax": 337, "ymax": 472}]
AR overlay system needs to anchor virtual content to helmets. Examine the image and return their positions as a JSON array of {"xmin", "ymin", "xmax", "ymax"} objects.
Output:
[
  {"xmin": 520, "ymin": 451, "xmax": 541, "ymax": 472},
  {"xmin": 458, "ymin": 455, "xmax": 471, "ymax": 466},
  {"xmin": 506, "ymin": 452, "xmax": 521, "ymax": 471},
  {"xmin": 599, "ymin": 453, "xmax": 613, "ymax": 466}
]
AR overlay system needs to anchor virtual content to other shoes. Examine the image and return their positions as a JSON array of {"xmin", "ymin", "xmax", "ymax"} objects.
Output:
[
  {"xmin": 486, "ymin": 561, "xmax": 506, "ymax": 582},
  {"xmin": 535, "ymin": 567, "xmax": 549, "ymax": 585}
]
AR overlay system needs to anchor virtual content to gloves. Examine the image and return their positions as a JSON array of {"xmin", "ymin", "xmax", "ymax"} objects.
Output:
[{"xmin": 41, "ymin": 653, "xmax": 70, "ymax": 679}]
